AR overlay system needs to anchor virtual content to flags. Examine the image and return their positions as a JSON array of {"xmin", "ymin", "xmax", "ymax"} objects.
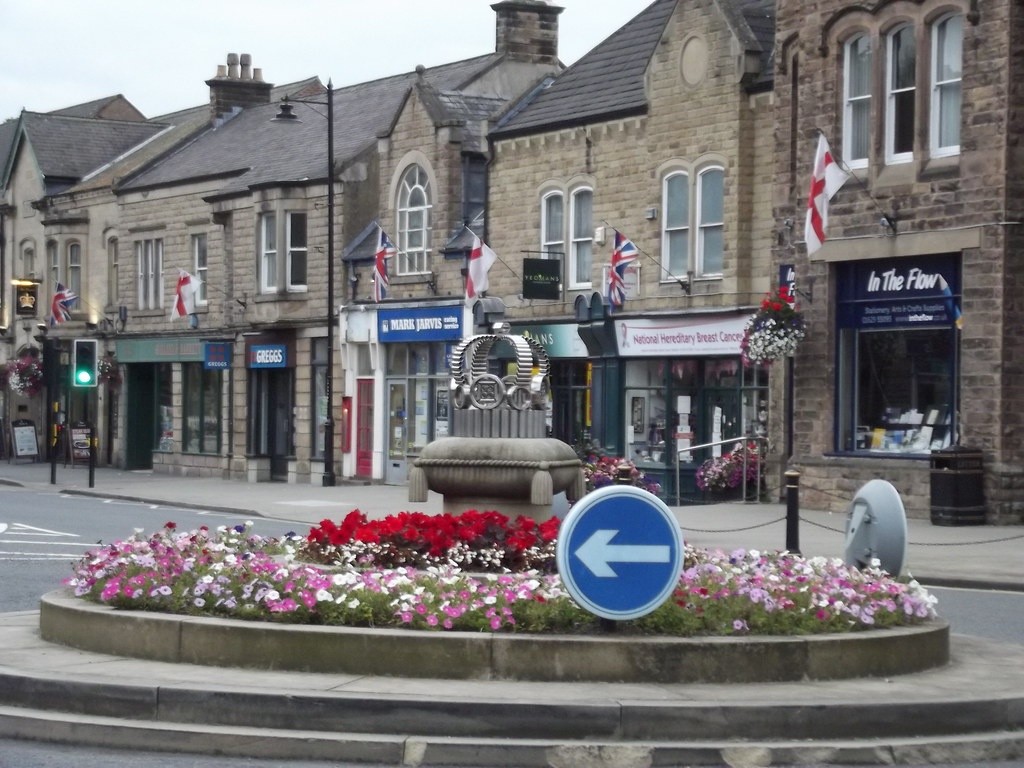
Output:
[
  {"xmin": 465, "ymin": 235, "xmax": 498, "ymax": 301},
  {"xmin": 805, "ymin": 132, "xmax": 851, "ymax": 256},
  {"xmin": 609, "ymin": 229, "xmax": 639, "ymax": 312},
  {"xmin": 50, "ymin": 282, "xmax": 78, "ymax": 326},
  {"xmin": 375, "ymin": 230, "xmax": 398, "ymax": 301},
  {"xmin": 171, "ymin": 270, "xmax": 203, "ymax": 321}
]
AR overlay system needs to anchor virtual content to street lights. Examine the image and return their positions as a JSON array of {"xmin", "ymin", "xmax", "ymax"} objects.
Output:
[
  {"xmin": 34, "ymin": 334, "xmax": 57, "ymax": 485},
  {"xmin": 271, "ymin": 96, "xmax": 336, "ymax": 486}
]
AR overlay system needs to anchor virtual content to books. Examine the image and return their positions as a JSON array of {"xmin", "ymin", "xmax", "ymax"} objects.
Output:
[{"xmin": 857, "ymin": 407, "xmax": 957, "ymax": 450}]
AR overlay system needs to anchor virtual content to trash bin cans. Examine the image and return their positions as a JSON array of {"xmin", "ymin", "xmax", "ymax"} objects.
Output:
[{"xmin": 928, "ymin": 445, "xmax": 986, "ymax": 527}]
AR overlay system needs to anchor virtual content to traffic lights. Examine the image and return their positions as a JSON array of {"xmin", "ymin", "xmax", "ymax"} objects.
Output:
[{"xmin": 73, "ymin": 339, "xmax": 98, "ymax": 387}]
